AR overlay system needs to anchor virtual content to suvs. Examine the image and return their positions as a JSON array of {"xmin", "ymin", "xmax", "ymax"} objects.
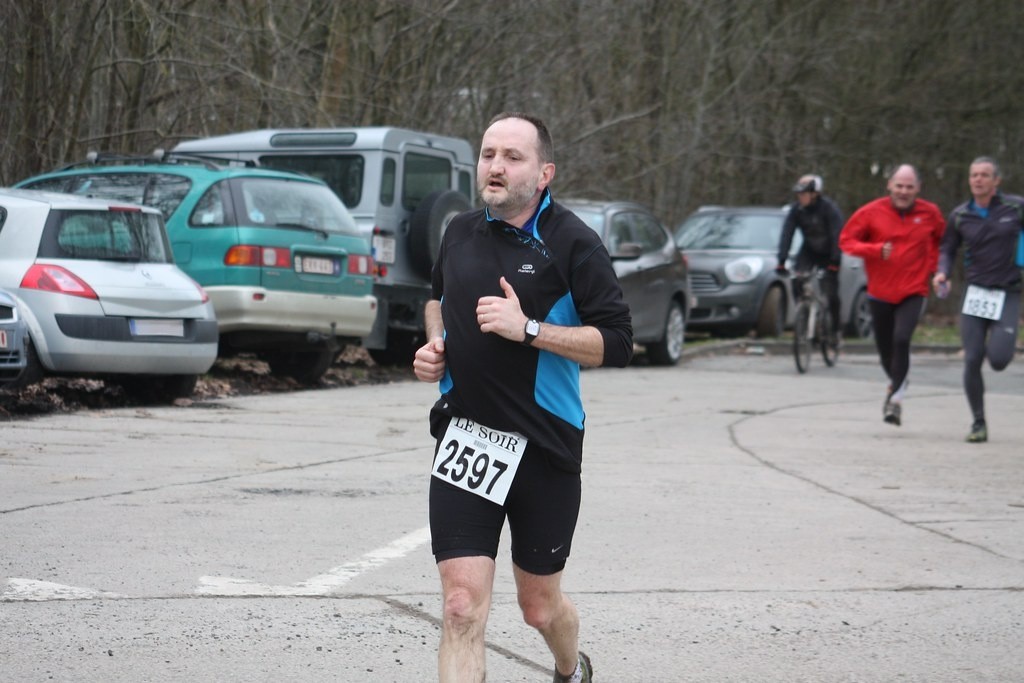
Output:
[{"xmin": 671, "ymin": 205, "xmax": 820, "ymax": 342}]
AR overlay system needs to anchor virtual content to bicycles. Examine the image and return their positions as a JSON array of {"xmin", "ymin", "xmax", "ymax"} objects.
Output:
[{"xmin": 782, "ymin": 266, "xmax": 840, "ymax": 374}]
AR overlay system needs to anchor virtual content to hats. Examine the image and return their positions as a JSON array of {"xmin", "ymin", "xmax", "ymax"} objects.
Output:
[{"xmin": 791, "ymin": 174, "xmax": 822, "ymax": 193}]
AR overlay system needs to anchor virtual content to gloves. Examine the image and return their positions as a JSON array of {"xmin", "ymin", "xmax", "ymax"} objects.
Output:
[
  {"xmin": 776, "ymin": 267, "xmax": 786, "ymax": 275},
  {"xmin": 826, "ymin": 271, "xmax": 836, "ymax": 280}
]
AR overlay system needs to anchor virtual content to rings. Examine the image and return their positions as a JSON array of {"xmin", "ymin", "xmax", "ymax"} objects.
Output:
[{"xmin": 482, "ymin": 314, "xmax": 483, "ymax": 321}]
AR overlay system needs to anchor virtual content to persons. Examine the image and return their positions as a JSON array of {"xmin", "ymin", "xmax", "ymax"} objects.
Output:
[
  {"xmin": 837, "ymin": 162, "xmax": 945, "ymax": 426},
  {"xmin": 412, "ymin": 112, "xmax": 634, "ymax": 683},
  {"xmin": 775, "ymin": 176, "xmax": 843, "ymax": 353},
  {"xmin": 932, "ymin": 155, "xmax": 1024, "ymax": 443}
]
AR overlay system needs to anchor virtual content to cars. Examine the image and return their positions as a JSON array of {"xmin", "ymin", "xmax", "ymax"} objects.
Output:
[
  {"xmin": 553, "ymin": 196, "xmax": 691, "ymax": 367},
  {"xmin": 10, "ymin": 144, "xmax": 377, "ymax": 385},
  {"xmin": 0, "ymin": 185, "xmax": 220, "ymax": 402},
  {"xmin": 839, "ymin": 252, "xmax": 929, "ymax": 339}
]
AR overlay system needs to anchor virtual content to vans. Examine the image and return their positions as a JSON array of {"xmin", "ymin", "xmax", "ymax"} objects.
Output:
[{"xmin": 162, "ymin": 124, "xmax": 476, "ymax": 369}]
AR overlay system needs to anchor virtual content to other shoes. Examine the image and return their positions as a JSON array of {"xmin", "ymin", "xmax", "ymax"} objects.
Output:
[
  {"xmin": 967, "ymin": 422, "xmax": 987, "ymax": 441},
  {"xmin": 552, "ymin": 651, "xmax": 593, "ymax": 683},
  {"xmin": 884, "ymin": 402, "xmax": 901, "ymax": 426}
]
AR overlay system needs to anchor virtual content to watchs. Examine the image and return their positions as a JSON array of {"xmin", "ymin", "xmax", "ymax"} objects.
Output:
[{"xmin": 523, "ymin": 318, "xmax": 540, "ymax": 346}]
